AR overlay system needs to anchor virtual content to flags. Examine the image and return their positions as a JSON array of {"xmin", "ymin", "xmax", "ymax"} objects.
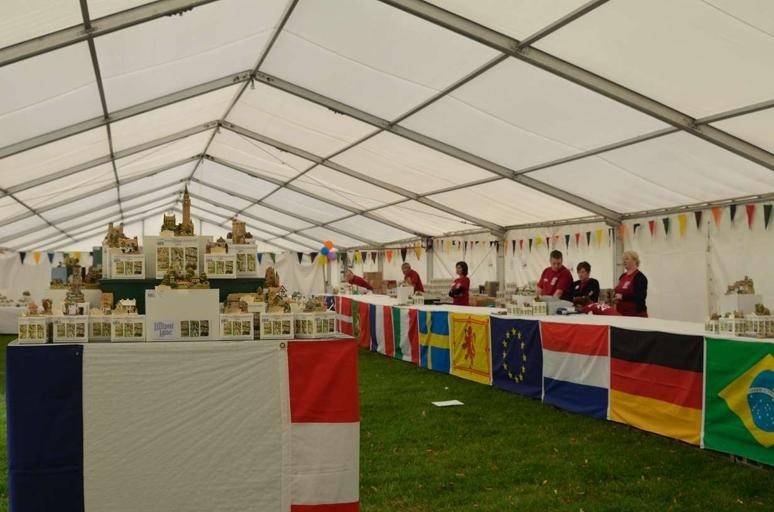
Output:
[
  {"xmin": 607, "ymin": 325, "xmax": 705, "ymax": 445},
  {"xmin": 327, "ymin": 296, "xmax": 354, "ymax": 336},
  {"xmin": 419, "ymin": 310, "xmax": 450, "ymax": 373},
  {"xmin": 448, "ymin": 312, "xmax": 493, "ymax": 386},
  {"xmin": 351, "ymin": 301, "xmax": 371, "ymax": 349},
  {"xmin": 489, "ymin": 316, "xmax": 542, "ymax": 399},
  {"xmin": 391, "ymin": 307, "xmax": 420, "ymax": 363},
  {"xmin": 540, "ymin": 321, "xmax": 609, "ymax": 417},
  {"xmin": 699, "ymin": 337, "xmax": 773, "ymax": 466},
  {"xmin": 369, "ymin": 303, "xmax": 395, "ymax": 357}
]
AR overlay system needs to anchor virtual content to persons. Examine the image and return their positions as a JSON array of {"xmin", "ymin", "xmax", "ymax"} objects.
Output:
[
  {"xmin": 402, "ymin": 263, "xmax": 425, "ymax": 294},
  {"xmin": 560, "ymin": 261, "xmax": 601, "ymax": 313},
  {"xmin": 449, "ymin": 262, "xmax": 470, "ymax": 305},
  {"xmin": 536, "ymin": 250, "xmax": 573, "ymax": 298},
  {"xmin": 614, "ymin": 250, "xmax": 648, "ymax": 317},
  {"xmin": 345, "ymin": 269, "xmax": 374, "ymax": 290}
]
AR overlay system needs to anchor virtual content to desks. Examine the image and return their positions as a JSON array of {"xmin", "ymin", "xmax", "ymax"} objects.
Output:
[
  {"xmin": 8, "ymin": 338, "xmax": 360, "ymax": 512},
  {"xmin": 339, "ymin": 292, "xmax": 773, "ymax": 467}
]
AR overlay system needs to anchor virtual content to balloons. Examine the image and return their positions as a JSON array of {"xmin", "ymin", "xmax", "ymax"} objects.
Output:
[{"xmin": 318, "ymin": 241, "xmax": 336, "ymax": 266}]
